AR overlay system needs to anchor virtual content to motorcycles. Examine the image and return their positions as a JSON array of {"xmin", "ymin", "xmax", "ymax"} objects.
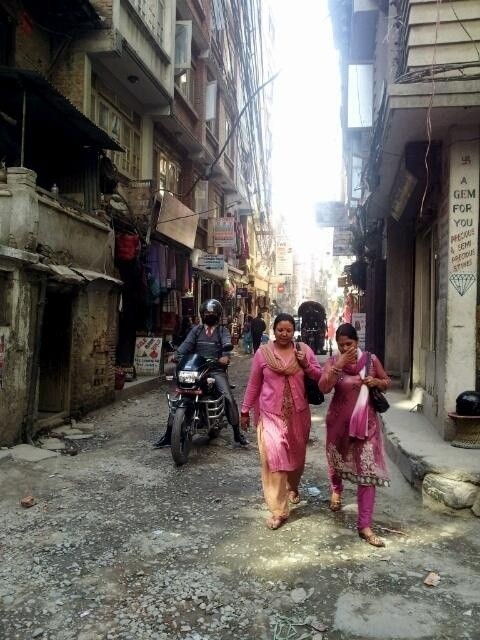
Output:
[{"xmin": 166, "ymin": 342, "xmax": 235, "ymax": 466}]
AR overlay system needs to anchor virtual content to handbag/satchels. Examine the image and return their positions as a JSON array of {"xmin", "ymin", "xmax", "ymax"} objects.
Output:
[
  {"xmin": 369, "ymin": 386, "xmax": 390, "ymax": 413},
  {"xmin": 304, "ymin": 374, "xmax": 325, "ymax": 405}
]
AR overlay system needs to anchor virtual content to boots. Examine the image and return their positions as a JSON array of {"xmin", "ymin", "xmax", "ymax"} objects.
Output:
[
  {"xmin": 232, "ymin": 424, "xmax": 250, "ymax": 445},
  {"xmin": 154, "ymin": 425, "xmax": 172, "ymax": 447}
]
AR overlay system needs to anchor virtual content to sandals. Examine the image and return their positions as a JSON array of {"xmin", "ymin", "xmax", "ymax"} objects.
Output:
[
  {"xmin": 266, "ymin": 516, "xmax": 288, "ymax": 530},
  {"xmin": 288, "ymin": 489, "xmax": 300, "ymax": 504}
]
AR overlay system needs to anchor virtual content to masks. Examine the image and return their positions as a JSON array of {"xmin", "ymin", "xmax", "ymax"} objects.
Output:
[{"xmin": 203, "ymin": 315, "xmax": 218, "ymax": 326}]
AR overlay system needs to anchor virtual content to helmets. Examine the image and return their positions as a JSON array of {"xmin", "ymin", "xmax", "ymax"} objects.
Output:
[{"xmin": 199, "ymin": 298, "xmax": 224, "ymax": 324}]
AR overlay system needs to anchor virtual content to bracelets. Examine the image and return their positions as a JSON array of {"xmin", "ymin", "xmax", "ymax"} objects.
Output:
[
  {"xmin": 241, "ymin": 412, "xmax": 250, "ymax": 417},
  {"xmin": 330, "ymin": 365, "xmax": 343, "ymax": 374},
  {"xmin": 382, "ymin": 377, "xmax": 389, "ymax": 391},
  {"xmin": 302, "ymin": 360, "xmax": 311, "ymax": 370}
]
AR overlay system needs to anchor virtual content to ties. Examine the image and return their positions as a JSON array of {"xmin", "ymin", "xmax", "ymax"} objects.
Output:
[{"xmin": 207, "ymin": 326, "xmax": 211, "ymax": 337}]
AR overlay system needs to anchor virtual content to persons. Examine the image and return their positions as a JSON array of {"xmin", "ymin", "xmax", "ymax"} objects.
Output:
[
  {"xmin": 244, "ymin": 314, "xmax": 254, "ymax": 354},
  {"xmin": 252, "ymin": 312, "xmax": 267, "ymax": 355},
  {"xmin": 152, "ymin": 298, "xmax": 250, "ymax": 448},
  {"xmin": 235, "ymin": 306, "xmax": 244, "ymax": 334},
  {"xmin": 192, "ymin": 315, "xmax": 201, "ymax": 327},
  {"xmin": 239, "ymin": 312, "xmax": 322, "ymax": 530},
  {"xmin": 316, "ymin": 321, "xmax": 392, "ymax": 548},
  {"xmin": 178, "ymin": 316, "xmax": 193, "ymax": 341}
]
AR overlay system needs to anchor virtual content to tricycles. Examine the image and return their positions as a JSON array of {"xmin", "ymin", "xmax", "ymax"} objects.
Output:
[{"xmin": 297, "ymin": 301, "xmax": 332, "ymax": 356}]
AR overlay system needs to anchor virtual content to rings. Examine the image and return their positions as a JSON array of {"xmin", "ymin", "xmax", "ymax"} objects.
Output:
[{"xmin": 368, "ymin": 379, "xmax": 371, "ymax": 382}]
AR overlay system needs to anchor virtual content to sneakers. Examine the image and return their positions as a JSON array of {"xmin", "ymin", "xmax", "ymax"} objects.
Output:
[
  {"xmin": 330, "ymin": 491, "xmax": 342, "ymax": 511},
  {"xmin": 359, "ymin": 528, "xmax": 385, "ymax": 547}
]
